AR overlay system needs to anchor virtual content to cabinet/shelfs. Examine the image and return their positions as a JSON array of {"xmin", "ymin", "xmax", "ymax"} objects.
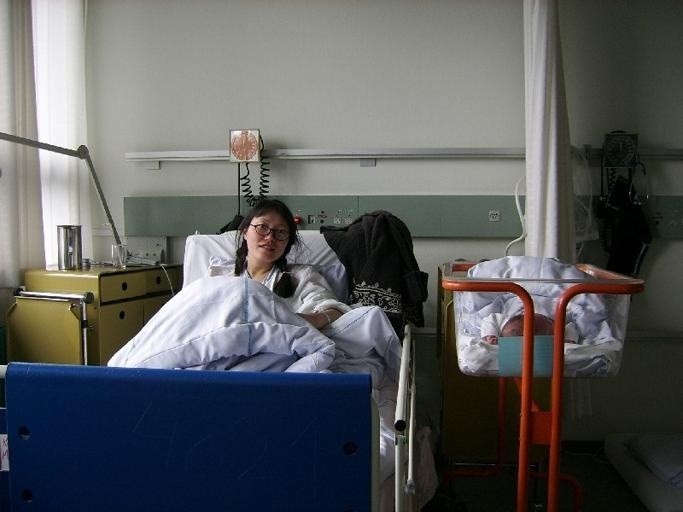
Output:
[{"xmin": 25, "ymin": 269, "xmax": 183, "ymax": 366}]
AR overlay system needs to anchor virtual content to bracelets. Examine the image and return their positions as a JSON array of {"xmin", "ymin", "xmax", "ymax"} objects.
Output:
[{"xmin": 319, "ymin": 311, "xmax": 331, "ymax": 324}]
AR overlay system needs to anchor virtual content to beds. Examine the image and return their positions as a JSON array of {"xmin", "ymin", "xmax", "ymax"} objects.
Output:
[{"xmin": 3, "ymin": 228, "xmax": 416, "ymax": 512}]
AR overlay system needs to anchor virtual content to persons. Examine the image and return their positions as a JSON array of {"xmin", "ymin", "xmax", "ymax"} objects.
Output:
[
  {"xmin": 224, "ymin": 200, "xmax": 352, "ymax": 329},
  {"xmin": 480, "ymin": 295, "xmax": 580, "ymax": 345}
]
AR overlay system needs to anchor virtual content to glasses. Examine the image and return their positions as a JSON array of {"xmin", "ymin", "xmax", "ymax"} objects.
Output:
[{"xmin": 249, "ymin": 223, "xmax": 290, "ymax": 241}]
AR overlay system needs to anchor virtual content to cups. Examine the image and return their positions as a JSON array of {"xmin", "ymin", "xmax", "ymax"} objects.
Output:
[
  {"xmin": 110, "ymin": 243, "xmax": 127, "ymax": 270},
  {"xmin": 56, "ymin": 224, "xmax": 83, "ymax": 270}
]
